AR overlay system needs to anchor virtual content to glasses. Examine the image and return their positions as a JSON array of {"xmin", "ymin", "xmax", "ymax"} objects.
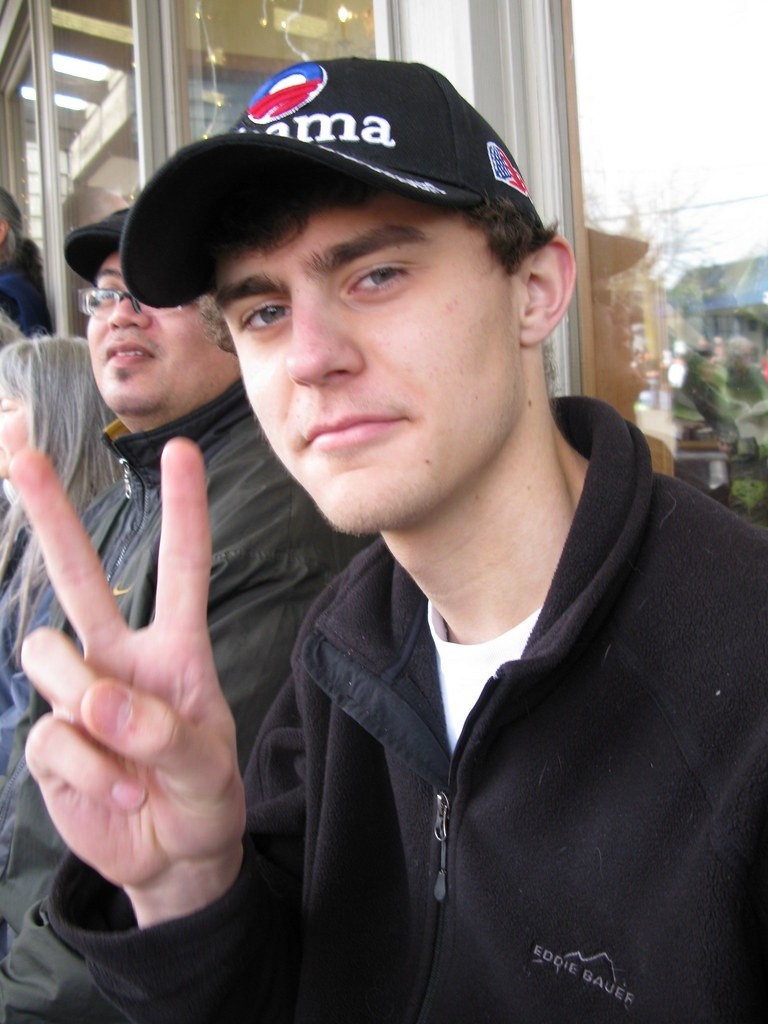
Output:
[{"xmin": 78, "ymin": 289, "xmax": 182, "ymax": 317}]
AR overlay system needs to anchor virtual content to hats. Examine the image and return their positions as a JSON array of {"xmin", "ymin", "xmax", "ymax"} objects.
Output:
[
  {"xmin": 62, "ymin": 210, "xmax": 138, "ymax": 284},
  {"xmin": 119, "ymin": 57, "xmax": 541, "ymax": 310}
]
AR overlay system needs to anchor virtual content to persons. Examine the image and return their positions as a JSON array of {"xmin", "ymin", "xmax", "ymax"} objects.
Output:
[
  {"xmin": 0, "ymin": 57, "xmax": 767, "ymax": 1024},
  {"xmin": 0, "ymin": 188, "xmax": 123, "ymax": 777},
  {"xmin": 579, "ymin": 226, "xmax": 767, "ymax": 477},
  {"xmin": 0, "ymin": 204, "xmax": 372, "ymax": 956}
]
